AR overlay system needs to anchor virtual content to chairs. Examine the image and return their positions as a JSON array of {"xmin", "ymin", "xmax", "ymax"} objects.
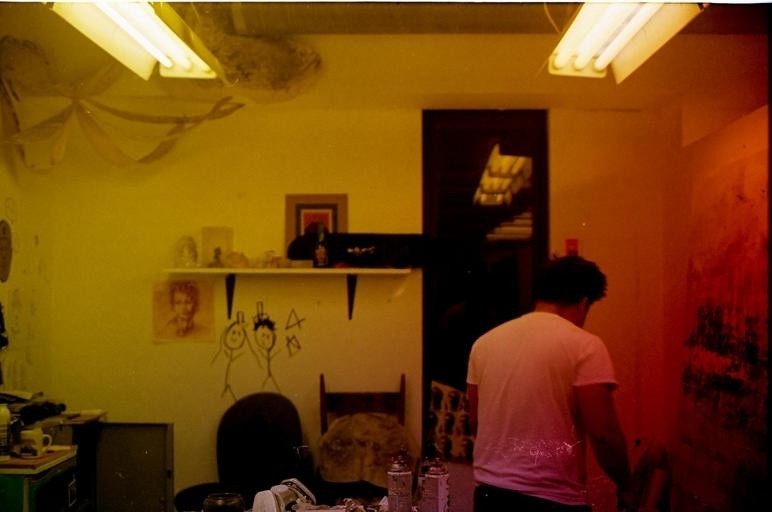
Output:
[{"xmin": 171, "ymin": 372, "xmax": 406, "ymax": 511}]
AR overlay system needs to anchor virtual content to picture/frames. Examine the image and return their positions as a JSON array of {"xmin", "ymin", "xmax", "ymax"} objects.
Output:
[{"xmin": 284, "ymin": 192, "xmax": 348, "ymax": 266}]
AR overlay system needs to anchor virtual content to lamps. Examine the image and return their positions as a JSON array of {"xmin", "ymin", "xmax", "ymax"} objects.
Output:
[
  {"xmin": 41, "ymin": 1, "xmax": 224, "ymax": 81},
  {"xmin": 546, "ymin": 2, "xmax": 703, "ymax": 85}
]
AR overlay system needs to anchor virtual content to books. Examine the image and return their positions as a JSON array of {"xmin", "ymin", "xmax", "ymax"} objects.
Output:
[{"xmin": 1, "ymin": 389, "xmax": 45, "ymax": 418}]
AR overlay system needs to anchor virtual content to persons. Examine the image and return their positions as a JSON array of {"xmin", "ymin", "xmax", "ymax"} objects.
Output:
[
  {"xmin": 464, "ymin": 255, "xmax": 645, "ymax": 512},
  {"xmin": 159, "ymin": 279, "xmax": 210, "ymax": 339}
]
